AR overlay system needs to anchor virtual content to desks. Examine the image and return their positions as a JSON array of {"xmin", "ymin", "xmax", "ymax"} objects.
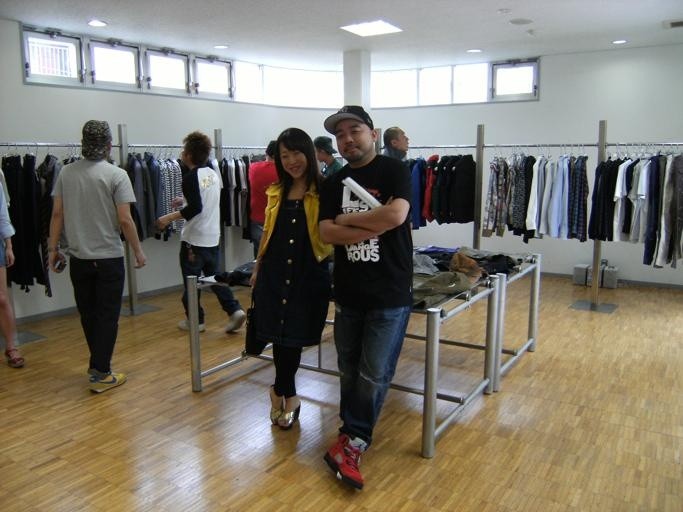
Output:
[
  {"xmin": 187, "ymin": 263, "xmax": 498, "ymax": 457},
  {"xmin": 319, "ymin": 247, "xmax": 540, "ymax": 393}
]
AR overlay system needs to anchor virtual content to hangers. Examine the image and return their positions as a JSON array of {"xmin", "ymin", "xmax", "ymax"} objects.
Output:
[
  {"xmin": 5, "ymin": 129, "xmax": 256, "ymax": 172},
  {"xmin": 410, "ymin": 140, "xmax": 681, "ymax": 165}
]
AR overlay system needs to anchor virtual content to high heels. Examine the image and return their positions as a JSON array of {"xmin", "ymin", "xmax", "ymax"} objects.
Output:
[{"xmin": 268, "ymin": 383, "xmax": 302, "ymax": 431}]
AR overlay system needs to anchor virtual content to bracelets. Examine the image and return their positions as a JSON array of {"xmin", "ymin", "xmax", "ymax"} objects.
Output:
[{"xmin": 46, "ymin": 247, "xmax": 60, "ymax": 252}]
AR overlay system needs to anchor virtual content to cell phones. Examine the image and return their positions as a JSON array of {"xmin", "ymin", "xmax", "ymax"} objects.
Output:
[{"xmin": 55, "ymin": 259, "xmax": 67, "ymax": 272}]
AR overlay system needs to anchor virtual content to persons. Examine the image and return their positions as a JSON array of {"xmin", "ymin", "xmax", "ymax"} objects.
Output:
[
  {"xmin": 246, "ymin": 139, "xmax": 279, "ymax": 259},
  {"xmin": 244, "ymin": 127, "xmax": 333, "ymax": 431},
  {"xmin": 317, "ymin": 103, "xmax": 414, "ymax": 493},
  {"xmin": 156, "ymin": 130, "xmax": 245, "ymax": 333},
  {"xmin": 313, "ymin": 136, "xmax": 342, "ymax": 177},
  {"xmin": 381, "ymin": 126, "xmax": 409, "ymax": 163},
  {"xmin": 44, "ymin": 119, "xmax": 145, "ymax": 393},
  {"xmin": 0, "ymin": 164, "xmax": 28, "ymax": 370}
]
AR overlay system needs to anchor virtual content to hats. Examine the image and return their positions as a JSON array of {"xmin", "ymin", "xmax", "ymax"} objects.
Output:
[
  {"xmin": 314, "ymin": 136, "xmax": 338, "ymax": 154},
  {"xmin": 81, "ymin": 119, "xmax": 113, "ymax": 162},
  {"xmin": 324, "ymin": 105, "xmax": 374, "ymax": 135}
]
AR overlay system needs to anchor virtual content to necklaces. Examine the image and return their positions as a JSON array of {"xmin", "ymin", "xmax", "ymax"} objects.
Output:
[{"xmin": 293, "ymin": 185, "xmax": 306, "ymax": 208}]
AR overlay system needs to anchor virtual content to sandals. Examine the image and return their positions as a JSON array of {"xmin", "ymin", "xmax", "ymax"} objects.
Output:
[{"xmin": 4, "ymin": 348, "xmax": 26, "ymax": 367}]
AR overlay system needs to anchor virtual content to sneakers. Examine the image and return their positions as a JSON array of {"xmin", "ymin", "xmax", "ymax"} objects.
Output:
[
  {"xmin": 87, "ymin": 368, "xmax": 128, "ymax": 394},
  {"xmin": 224, "ymin": 310, "xmax": 247, "ymax": 334},
  {"xmin": 324, "ymin": 432, "xmax": 369, "ymax": 491},
  {"xmin": 178, "ymin": 320, "xmax": 206, "ymax": 333}
]
